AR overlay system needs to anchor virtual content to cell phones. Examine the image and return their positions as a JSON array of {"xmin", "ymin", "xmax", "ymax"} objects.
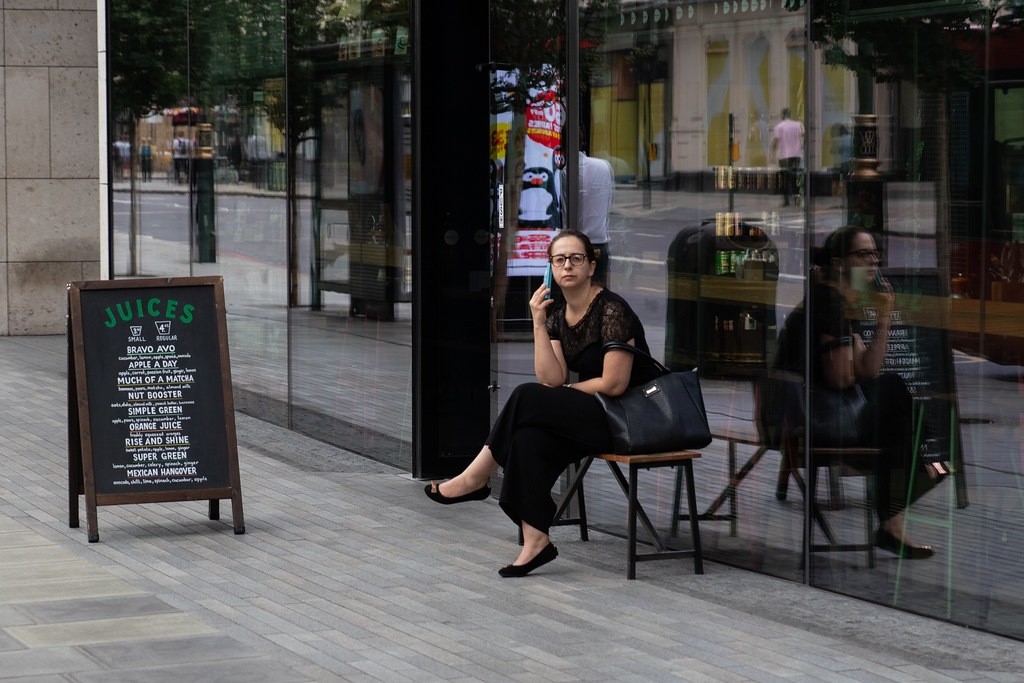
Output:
[
  {"xmin": 867, "ymin": 268, "xmax": 890, "ymax": 305},
  {"xmin": 543, "ymin": 263, "xmax": 552, "ymax": 300}
]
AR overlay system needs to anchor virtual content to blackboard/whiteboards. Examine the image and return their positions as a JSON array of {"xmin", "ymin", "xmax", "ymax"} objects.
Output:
[
  {"xmin": 66, "ymin": 275, "xmax": 242, "ymax": 505},
  {"xmin": 779, "ymin": 264, "xmax": 965, "ymax": 467}
]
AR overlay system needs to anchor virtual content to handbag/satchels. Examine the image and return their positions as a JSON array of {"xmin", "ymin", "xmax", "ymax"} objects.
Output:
[
  {"xmin": 756, "ymin": 335, "xmax": 870, "ymax": 450},
  {"xmin": 594, "ymin": 341, "xmax": 712, "ymax": 456}
]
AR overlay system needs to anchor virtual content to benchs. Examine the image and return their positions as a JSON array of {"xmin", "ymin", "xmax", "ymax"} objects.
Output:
[
  {"xmin": 671, "ymin": 423, "xmax": 881, "ymax": 587},
  {"xmin": 517, "ymin": 451, "xmax": 705, "ymax": 580}
]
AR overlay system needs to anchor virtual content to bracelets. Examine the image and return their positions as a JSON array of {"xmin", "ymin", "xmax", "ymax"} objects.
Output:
[{"xmin": 567, "ymin": 384, "xmax": 571, "ymax": 387}]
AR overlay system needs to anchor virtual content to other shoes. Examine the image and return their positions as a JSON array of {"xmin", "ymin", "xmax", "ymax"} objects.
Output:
[
  {"xmin": 876, "ymin": 529, "xmax": 933, "ymax": 559},
  {"xmin": 498, "ymin": 542, "xmax": 559, "ymax": 577},
  {"xmin": 425, "ymin": 481, "xmax": 491, "ymax": 505}
]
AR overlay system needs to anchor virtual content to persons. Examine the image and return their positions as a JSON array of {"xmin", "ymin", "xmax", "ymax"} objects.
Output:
[
  {"xmin": 771, "ymin": 107, "xmax": 850, "ymax": 209},
  {"xmin": 114, "ymin": 124, "xmax": 270, "ymax": 190},
  {"xmin": 553, "ymin": 129, "xmax": 614, "ymax": 293},
  {"xmin": 425, "ymin": 228, "xmax": 653, "ymax": 577},
  {"xmin": 764, "ymin": 225, "xmax": 951, "ymax": 557}
]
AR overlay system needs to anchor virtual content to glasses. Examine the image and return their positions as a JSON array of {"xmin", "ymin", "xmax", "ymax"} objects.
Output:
[
  {"xmin": 549, "ymin": 253, "xmax": 589, "ymax": 267},
  {"xmin": 848, "ymin": 249, "xmax": 879, "ymax": 261}
]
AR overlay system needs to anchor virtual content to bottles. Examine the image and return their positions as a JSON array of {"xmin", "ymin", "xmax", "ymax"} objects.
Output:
[
  {"xmin": 715, "ymin": 211, "xmax": 780, "ymax": 236},
  {"xmin": 715, "ymin": 249, "xmax": 776, "ymax": 276},
  {"xmin": 713, "ymin": 166, "xmax": 778, "ymax": 191}
]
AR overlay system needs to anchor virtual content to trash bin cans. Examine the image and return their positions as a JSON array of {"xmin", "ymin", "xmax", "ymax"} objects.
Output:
[{"xmin": 665, "ymin": 223, "xmax": 779, "ymax": 380}]
